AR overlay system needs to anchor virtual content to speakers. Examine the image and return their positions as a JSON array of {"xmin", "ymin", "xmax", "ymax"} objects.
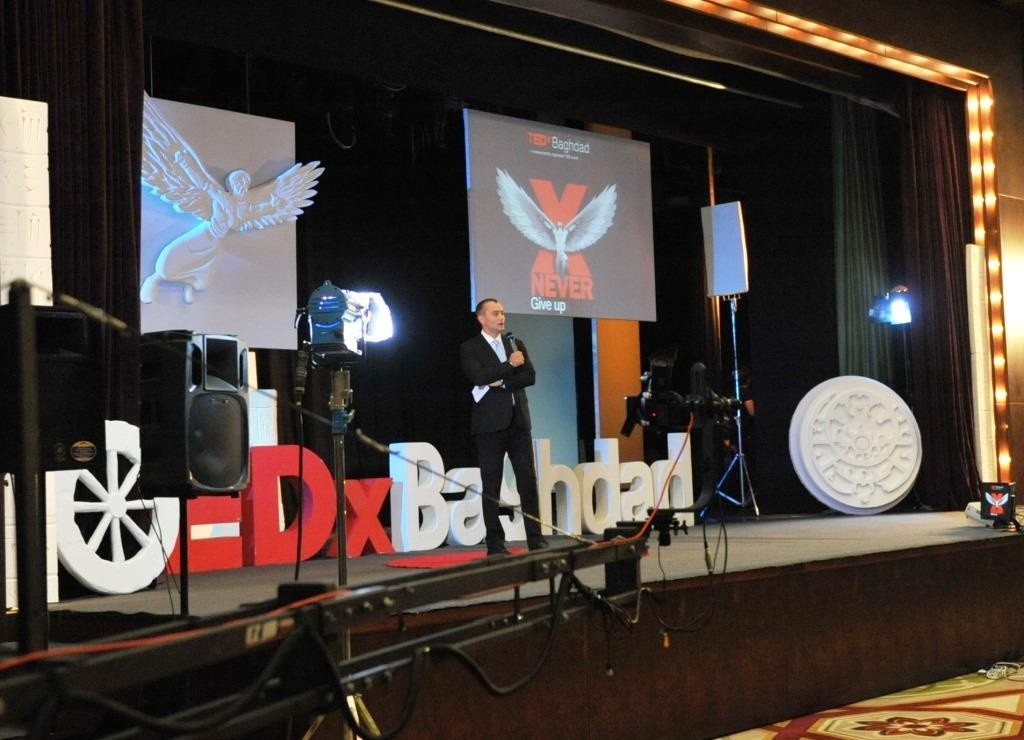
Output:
[
  {"xmin": 0, "ymin": 303, "xmax": 108, "ymax": 474},
  {"xmin": 700, "ymin": 201, "xmax": 750, "ymax": 298},
  {"xmin": 138, "ymin": 330, "xmax": 250, "ymax": 500}
]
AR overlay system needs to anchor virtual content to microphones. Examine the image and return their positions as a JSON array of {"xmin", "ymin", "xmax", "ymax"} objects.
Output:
[
  {"xmin": 293, "ymin": 351, "xmax": 310, "ymax": 405},
  {"xmin": 505, "ymin": 331, "xmax": 519, "ymax": 353}
]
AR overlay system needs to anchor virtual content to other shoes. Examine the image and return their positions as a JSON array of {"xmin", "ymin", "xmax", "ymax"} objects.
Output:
[
  {"xmin": 488, "ymin": 549, "xmax": 511, "ymax": 555},
  {"xmin": 529, "ymin": 542, "xmax": 548, "ymax": 549}
]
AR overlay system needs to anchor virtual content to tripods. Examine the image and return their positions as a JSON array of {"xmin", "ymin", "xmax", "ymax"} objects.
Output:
[{"xmin": 699, "ymin": 299, "xmax": 761, "ymax": 522}]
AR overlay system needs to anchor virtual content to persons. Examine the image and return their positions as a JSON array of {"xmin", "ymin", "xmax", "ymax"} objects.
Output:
[
  {"xmin": 458, "ymin": 299, "xmax": 548, "ymax": 556},
  {"xmin": 139, "ymin": 148, "xmax": 283, "ymax": 306},
  {"xmin": 717, "ymin": 364, "xmax": 756, "ymax": 518}
]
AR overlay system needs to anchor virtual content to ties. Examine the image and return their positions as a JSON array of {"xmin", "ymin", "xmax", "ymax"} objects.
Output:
[{"xmin": 493, "ymin": 339, "xmax": 505, "ymax": 363}]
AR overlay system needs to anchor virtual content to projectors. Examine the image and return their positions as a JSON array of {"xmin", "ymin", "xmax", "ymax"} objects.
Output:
[{"xmin": 965, "ymin": 501, "xmax": 1009, "ymax": 528}]
